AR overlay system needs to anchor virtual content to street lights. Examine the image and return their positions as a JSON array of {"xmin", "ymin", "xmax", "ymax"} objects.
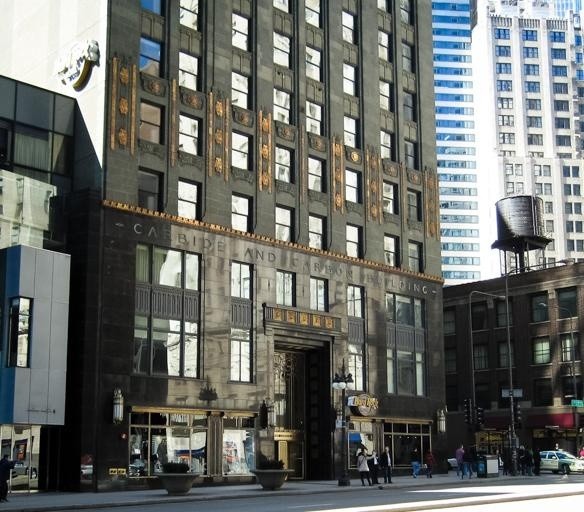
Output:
[
  {"xmin": 504, "ymin": 259, "xmax": 575, "ymax": 434},
  {"xmin": 539, "ymin": 303, "xmax": 580, "ymax": 455},
  {"xmin": 469, "ymin": 291, "xmax": 505, "ymax": 431}
]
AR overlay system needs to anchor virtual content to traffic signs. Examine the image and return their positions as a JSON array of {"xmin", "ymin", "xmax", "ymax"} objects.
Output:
[{"xmin": 571, "ymin": 399, "xmax": 583, "ymax": 407}]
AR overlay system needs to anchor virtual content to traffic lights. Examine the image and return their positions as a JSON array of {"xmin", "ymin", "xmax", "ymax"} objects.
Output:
[
  {"xmin": 476, "ymin": 407, "xmax": 484, "ymax": 425},
  {"xmin": 463, "ymin": 398, "xmax": 472, "ymax": 424},
  {"xmin": 513, "ymin": 403, "xmax": 520, "ymax": 424}
]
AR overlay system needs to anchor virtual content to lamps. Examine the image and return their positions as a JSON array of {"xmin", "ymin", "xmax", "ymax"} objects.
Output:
[
  {"xmin": 335, "ymin": 408, "xmax": 343, "ymax": 429},
  {"xmin": 113, "ymin": 388, "xmax": 125, "ymax": 425},
  {"xmin": 265, "ymin": 397, "xmax": 276, "ymax": 427},
  {"xmin": 437, "ymin": 409, "xmax": 446, "ymax": 432}
]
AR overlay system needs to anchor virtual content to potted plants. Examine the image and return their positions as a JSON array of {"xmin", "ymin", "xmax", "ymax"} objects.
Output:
[
  {"xmin": 153, "ymin": 463, "xmax": 200, "ymax": 496},
  {"xmin": 250, "ymin": 460, "xmax": 295, "ymax": 490}
]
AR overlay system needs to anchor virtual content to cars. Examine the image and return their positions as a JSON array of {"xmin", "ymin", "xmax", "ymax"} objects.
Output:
[{"xmin": 539, "ymin": 451, "xmax": 584, "ymax": 474}]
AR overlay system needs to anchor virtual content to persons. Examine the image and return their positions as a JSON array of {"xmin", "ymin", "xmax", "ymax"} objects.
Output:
[
  {"xmin": 579, "ymin": 445, "xmax": 584, "ymax": 458},
  {"xmin": 157, "ymin": 439, "xmax": 167, "ymax": 465},
  {"xmin": 408, "ymin": 448, "xmax": 420, "ymax": 478},
  {"xmin": 367, "ymin": 450, "xmax": 379, "ymax": 485},
  {"xmin": 130, "ymin": 436, "xmax": 139, "ymax": 453},
  {"xmin": 0, "ymin": 454, "xmax": 15, "ymax": 503},
  {"xmin": 357, "ymin": 448, "xmax": 372, "ymax": 486},
  {"xmin": 380, "ymin": 446, "xmax": 392, "ymax": 484},
  {"xmin": 425, "ymin": 449, "xmax": 436, "ymax": 478},
  {"xmin": 464, "ymin": 451, "xmax": 473, "ymax": 478},
  {"xmin": 555, "ymin": 443, "xmax": 560, "ymax": 451},
  {"xmin": 455, "ymin": 443, "xmax": 465, "ymax": 479},
  {"xmin": 519, "ymin": 445, "xmax": 540, "ymax": 477},
  {"xmin": 500, "ymin": 448, "xmax": 508, "ymax": 475}
]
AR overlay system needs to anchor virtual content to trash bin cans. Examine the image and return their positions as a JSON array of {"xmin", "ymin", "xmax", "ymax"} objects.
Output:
[{"xmin": 476, "ymin": 454, "xmax": 499, "ymax": 477}]
